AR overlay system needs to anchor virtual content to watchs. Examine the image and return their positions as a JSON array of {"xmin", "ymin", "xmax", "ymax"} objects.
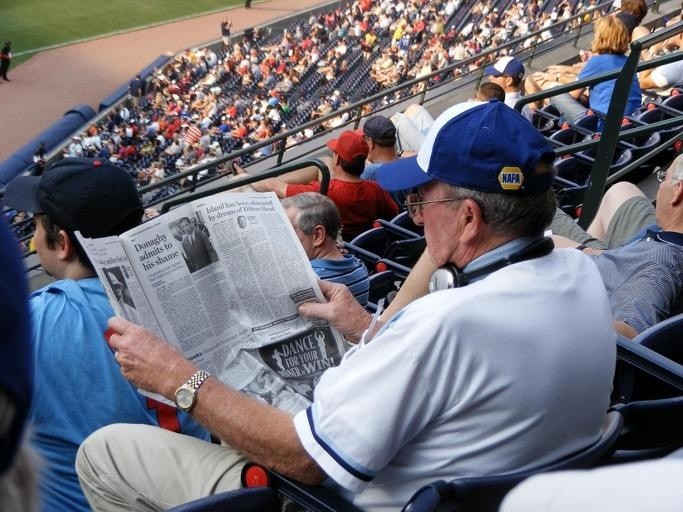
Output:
[{"xmin": 173, "ymin": 370, "xmax": 211, "ymax": 415}]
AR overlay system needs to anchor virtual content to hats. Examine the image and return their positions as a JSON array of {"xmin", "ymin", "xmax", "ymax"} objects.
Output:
[
  {"xmin": 1, "ymin": 152, "xmax": 143, "ymax": 238},
  {"xmin": 485, "ymin": 55, "xmax": 525, "ymax": 79},
  {"xmin": 326, "ymin": 115, "xmax": 397, "ymax": 163},
  {"xmin": 375, "ymin": 97, "xmax": 556, "ymax": 195}
]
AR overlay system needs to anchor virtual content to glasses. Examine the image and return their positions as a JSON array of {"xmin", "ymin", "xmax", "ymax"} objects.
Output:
[
  {"xmin": 402, "ymin": 187, "xmax": 468, "ymax": 220},
  {"xmin": 657, "ymin": 170, "xmax": 683, "ymax": 182}
]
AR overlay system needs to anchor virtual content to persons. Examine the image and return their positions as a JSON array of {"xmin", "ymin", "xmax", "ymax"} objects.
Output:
[
  {"xmin": 73, "ymin": 99, "xmax": 615, "ymax": 512},
  {"xmin": 1, "ymin": 0, "xmax": 683, "ymax": 341},
  {"xmin": 1, "ymin": 155, "xmax": 213, "ymax": 512}
]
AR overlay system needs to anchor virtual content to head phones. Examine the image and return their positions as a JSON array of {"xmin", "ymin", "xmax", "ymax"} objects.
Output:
[{"xmin": 428, "ymin": 236, "xmax": 555, "ymax": 293}]
